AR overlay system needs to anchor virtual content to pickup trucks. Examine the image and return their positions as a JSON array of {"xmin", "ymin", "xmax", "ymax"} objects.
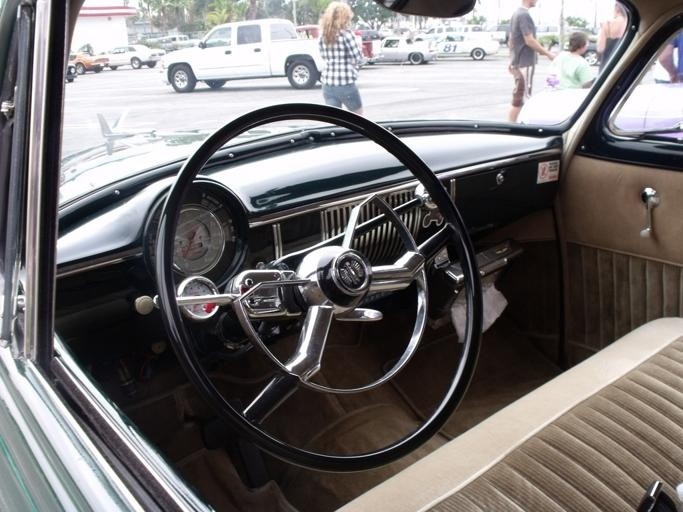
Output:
[
  {"xmin": 294, "ymin": 25, "xmax": 374, "ymax": 68},
  {"xmin": 160, "ymin": 19, "xmax": 363, "ymax": 92}
]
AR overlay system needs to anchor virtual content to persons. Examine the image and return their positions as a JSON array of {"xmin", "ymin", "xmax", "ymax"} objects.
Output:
[
  {"xmin": 318, "ymin": 0, "xmax": 368, "ymax": 116},
  {"xmin": 507, "ymin": 0, "xmax": 555, "ymax": 125},
  {"xmin": 597, "ymin": 2, "xmax": 627, "ymax": 74},
  {"xmin": 546, "ymin": 31, "xmax": 597, "ymax": 89},
  {"xmin": 659, "ymin": 31, "xmax": 683, "ymax": 83}
]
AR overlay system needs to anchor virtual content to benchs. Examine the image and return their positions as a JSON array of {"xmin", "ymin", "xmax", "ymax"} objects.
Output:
[{"xmin": 330, "ymin": 313, "xmax": 681, "ymax": 511}]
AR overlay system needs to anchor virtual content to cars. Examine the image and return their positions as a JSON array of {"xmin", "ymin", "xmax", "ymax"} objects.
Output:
[
  {"xmin": 62, "ymin": 33, "xmax": 198, "ymax": 85},
  {"xmin": 349, "ymin": 20, "xmax": 604, "ymax": 65},
  {"xmin": 0, "ymin": 1, "xmax": 683, "ymax": 510}
]
